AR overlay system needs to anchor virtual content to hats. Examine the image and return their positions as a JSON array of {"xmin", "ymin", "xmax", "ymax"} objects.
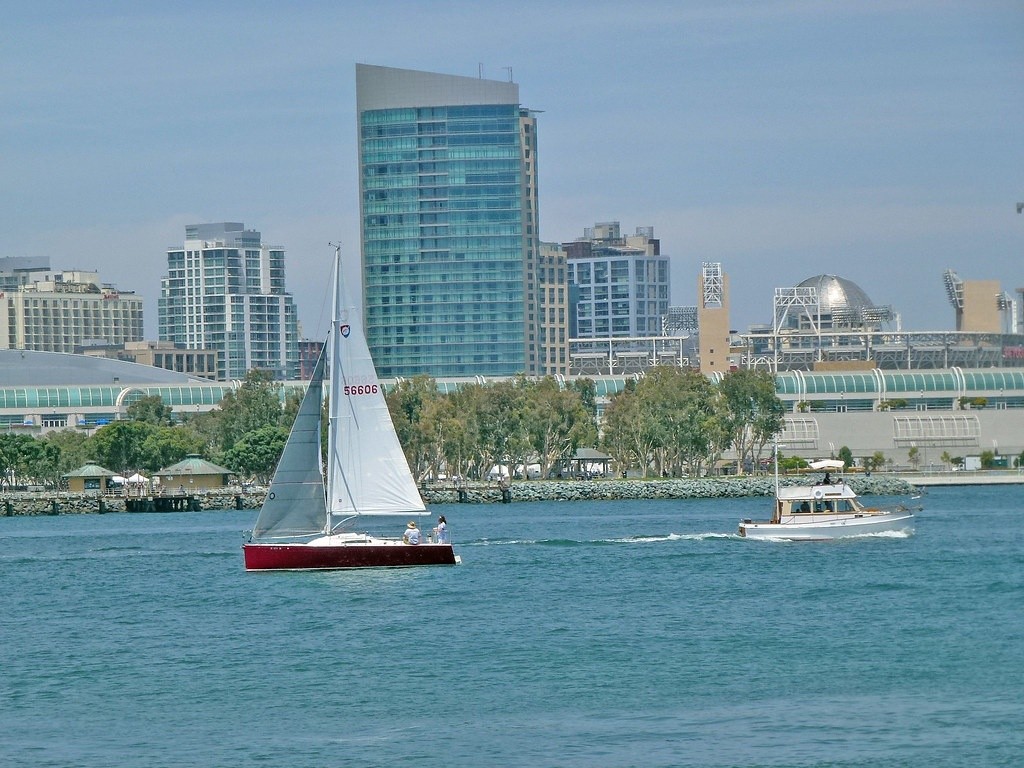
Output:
[{"xmin": 406, "ymin": 521, "xmax": 416, "ymax": 529}]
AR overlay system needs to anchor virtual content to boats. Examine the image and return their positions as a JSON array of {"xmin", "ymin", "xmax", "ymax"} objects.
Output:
[{"xmin": 739, "ymin": 451, "xmax": 926, "ymax": 537}]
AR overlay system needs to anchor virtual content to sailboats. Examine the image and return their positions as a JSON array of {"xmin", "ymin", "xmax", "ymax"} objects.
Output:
[{"xmin": 241, "ymin": 238, "xmax": 462, "ymax": 567}]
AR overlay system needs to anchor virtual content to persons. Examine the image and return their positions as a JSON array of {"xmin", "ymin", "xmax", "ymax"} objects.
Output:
[
  {"xmin": 575, "ymin": 468, "xmax": 605, "ymax": 480},
  {"xmin": 178, "ymin": 483, "xmax": 186, "ymax": 495},
  {"xmin": 662, "ymin": 468, "xmax": 668, "ymax": 477},
  {"xmin": 403, "ymin": 520, "xmax": 421, "ymax": 545},
  {"xmin": 823, "ymin": 473, "xmax": 834, "ymax": 484},
  {"xmin": 622, "ymin": 469, "xmax": 627, "ymax": 478},
  {"xmin": 434, "ymin": 515, "xmax": 447, "ymax": 544},
  {"xmin": 454, "ymin": 471, "xmax": 505, "ymax": 490}
]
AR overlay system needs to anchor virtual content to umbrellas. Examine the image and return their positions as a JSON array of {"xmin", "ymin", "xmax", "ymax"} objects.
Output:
[
  {"xmin": 112, "ymin": 475, "xmax": 126, "ymax": 484},
  {"xmin": 124, "ymin": 472, "xmax": 150, "ymax": 483}
]
